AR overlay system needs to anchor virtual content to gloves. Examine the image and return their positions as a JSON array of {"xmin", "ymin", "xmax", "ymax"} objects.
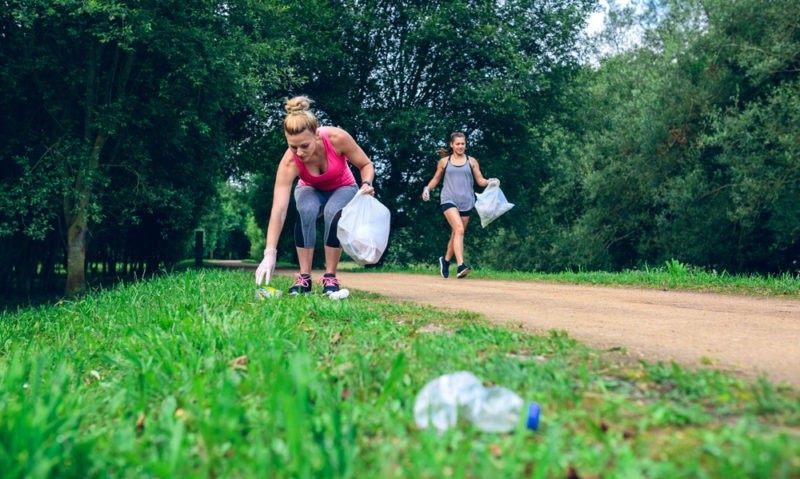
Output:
[
  {"xmin": 255, "ymin": 247, "xmax": 277, "ymax": 286},
  {"xmin": 421, "ymin": 186, "xmax": 430, "ymax": 202},
  {"xmin": 486, "ymin": 177, "xmax": 500, "ymax": 186}
]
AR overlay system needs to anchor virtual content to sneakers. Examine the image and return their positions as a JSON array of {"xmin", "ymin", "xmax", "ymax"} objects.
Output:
[
  {"xmin": 321, "ymin": 273, "xmax": 340, "ymax": 296},
  {"xmin": 439, "ymin": 256, "xmax": 451, "ymax": 279},
  {"xmin": 287, "ymin": 273, "xmax": 312, "ymax": 295},
  {"xmin": 456, "ymin": 262, "xmax": 471, "ymax": 278}
]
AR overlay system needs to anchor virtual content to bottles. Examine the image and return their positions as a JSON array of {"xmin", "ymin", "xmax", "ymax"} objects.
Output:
[{"xmin": 414, "ymin": 370, "xmax": 542, "ymax": 436}]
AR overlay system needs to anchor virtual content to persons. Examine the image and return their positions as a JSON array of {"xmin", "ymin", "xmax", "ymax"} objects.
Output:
[
  {"xmin": 421, "ymin": 132, "xmax": 501, "ymax": 279},
  {"xmin": 255, "ymin": 95, "xmax": 374, "ymax": 298}
]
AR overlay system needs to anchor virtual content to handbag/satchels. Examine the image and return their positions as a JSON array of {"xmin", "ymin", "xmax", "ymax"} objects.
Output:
[
  {"xmin": 474, "ymin": 180, "xmax": 516, "ymax": 230},
  {"xmin": 336, "ymin": 188, "xmax": 391, "ymax": 266}
]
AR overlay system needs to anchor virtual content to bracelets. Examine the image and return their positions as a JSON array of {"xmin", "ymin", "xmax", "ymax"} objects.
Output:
[{"xmin": 362, "ymin": 181, "xmax": 372, "ymax": 186}]
